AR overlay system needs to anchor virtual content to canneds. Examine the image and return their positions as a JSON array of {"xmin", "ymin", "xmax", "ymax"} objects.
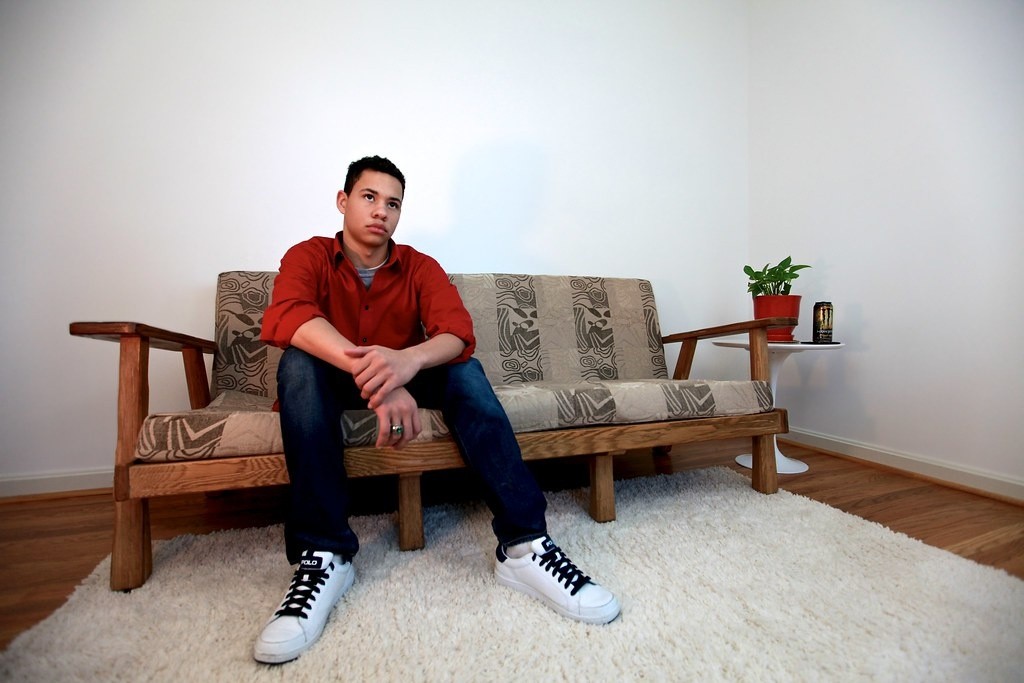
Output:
[{"xmin": 812, "ymin": 301, "xmax": 833, "ymax": 342}]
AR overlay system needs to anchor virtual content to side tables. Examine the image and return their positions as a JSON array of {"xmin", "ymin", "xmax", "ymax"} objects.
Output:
[{"xmin": 716, "ymin": 337, "xmax": 845, "ymax": 474}]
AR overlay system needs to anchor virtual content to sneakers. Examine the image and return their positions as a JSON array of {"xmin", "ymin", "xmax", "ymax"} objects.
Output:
[
  {"xmin": 493, "ymin": 534, "xmax": 622, "ymax": 624},
  {"xmin": 252, "ymin": 549, "xmax": 354, "ymax": 665}
]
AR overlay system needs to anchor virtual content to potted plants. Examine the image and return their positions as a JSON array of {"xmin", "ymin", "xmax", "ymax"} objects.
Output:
[{"xmin": 744, "ymin": 254, "xmax": 814, "ymax": 345}]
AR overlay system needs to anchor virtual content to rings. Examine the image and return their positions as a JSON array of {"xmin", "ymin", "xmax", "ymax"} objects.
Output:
[{"xmin": 390, "ymin": 424, "xmax": 404, "ymax": 435}]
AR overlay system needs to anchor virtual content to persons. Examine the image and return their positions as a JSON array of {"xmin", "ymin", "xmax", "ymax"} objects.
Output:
[{"xmin": 253, "ymin": 155, "xmax": 622, "ymax": 666}]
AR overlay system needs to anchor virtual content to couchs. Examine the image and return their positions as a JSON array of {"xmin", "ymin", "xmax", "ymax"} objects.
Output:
[{"xmin": 67, "ymin": 270, "xmax": 792, "ymax": 592}]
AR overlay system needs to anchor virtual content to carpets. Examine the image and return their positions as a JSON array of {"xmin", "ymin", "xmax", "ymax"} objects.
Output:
[{"xmin": 0, "ymin": 465, "xmax": 1024, "ymax": 683}]
